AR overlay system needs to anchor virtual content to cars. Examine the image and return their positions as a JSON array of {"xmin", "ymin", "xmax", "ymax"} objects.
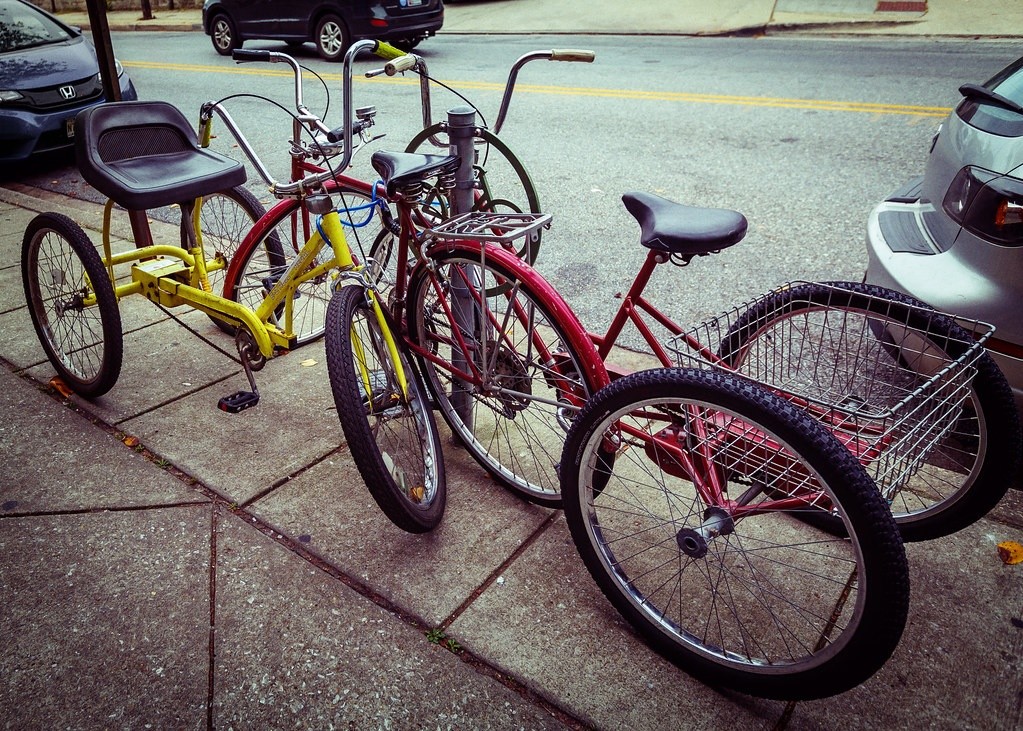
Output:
[
  {"xmin": 201, "ymin": 0, "xmax": 446, "ymax": 64},
  {"xmin": 860, "ymin": 51, "xmax": 1023, "ymax": 486},
  {"xmin": 0, "ymin": 0, "xmax": 138, "ymax": 185}
]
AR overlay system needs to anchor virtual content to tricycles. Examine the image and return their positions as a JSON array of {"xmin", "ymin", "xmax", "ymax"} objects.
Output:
[
  {"xmin": 19, "ymin": 35, "xmax": 448, "ymax": 538},
  {"xmin": 364, "ymin": 45, "xmax": 1023, "ymax": 705}
]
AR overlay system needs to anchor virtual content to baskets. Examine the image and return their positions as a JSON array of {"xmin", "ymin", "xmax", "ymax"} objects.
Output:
[{"xmin": 665, "ymin": 278, "xmax": 995, "ymax": 526}]
